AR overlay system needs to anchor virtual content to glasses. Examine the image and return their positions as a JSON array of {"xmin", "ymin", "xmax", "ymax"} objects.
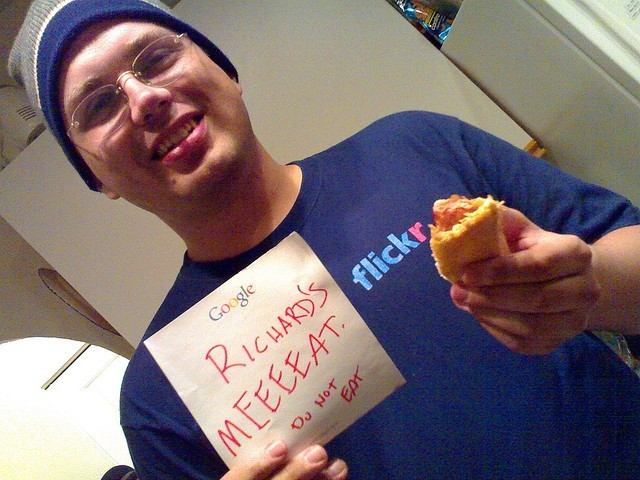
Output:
[{"xmin": 63, "ymin": 29, "xmax": 190, "ymax": 144}]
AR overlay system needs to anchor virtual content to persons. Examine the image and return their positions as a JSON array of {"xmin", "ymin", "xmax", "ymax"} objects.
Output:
[{"xmin": 6, "ymin": 1, "xmax": 640, "ymax": 480}]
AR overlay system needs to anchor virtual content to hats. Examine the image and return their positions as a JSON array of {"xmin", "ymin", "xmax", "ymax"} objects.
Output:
[{"xmin": 6, "ymin": 1, "xmax": 240, "ymax": 194}]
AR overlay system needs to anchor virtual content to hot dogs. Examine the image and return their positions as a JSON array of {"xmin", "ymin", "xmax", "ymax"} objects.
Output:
[{"xmin": 428, "ymin": 194, "xmax": 511, "ymax": 283}]
